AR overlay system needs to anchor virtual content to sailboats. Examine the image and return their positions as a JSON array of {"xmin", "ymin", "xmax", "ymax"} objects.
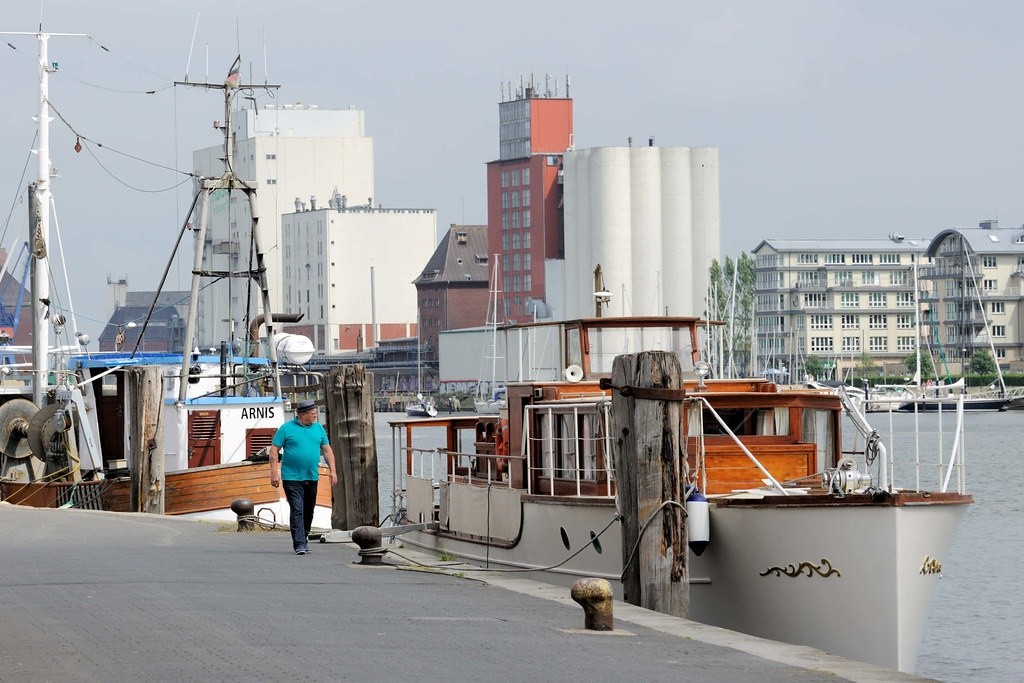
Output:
[
  {"xmin": 406, "ymin": 307, "xmax": 438, "ymax": 417},
  {"xmin": 0, "ymin": 13, "xmax": 284, "ymax": 515},
  {"xmin": 474, "ymin": 234, "xmax": 1010, "ymax": 413}
]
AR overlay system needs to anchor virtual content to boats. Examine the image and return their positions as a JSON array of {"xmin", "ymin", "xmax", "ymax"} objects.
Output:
[{"xmin": 386, "ymin": 314, "xmax": 976, "ymax": 673}]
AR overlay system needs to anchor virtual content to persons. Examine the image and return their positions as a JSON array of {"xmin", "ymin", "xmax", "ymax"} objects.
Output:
[{"xmin": 270, "ymin": 399, "xmax": 338, "ymax": 554}]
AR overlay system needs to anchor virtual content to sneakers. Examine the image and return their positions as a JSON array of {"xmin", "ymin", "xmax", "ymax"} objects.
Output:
[{"xmin": 296, "ymin": 548, "xmax": 312, "ymax": 555}]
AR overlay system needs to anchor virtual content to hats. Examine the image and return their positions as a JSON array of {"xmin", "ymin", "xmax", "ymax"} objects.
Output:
[{"xmin": 296, "ymin": 399, "xmax": 318, "ymax": 413}]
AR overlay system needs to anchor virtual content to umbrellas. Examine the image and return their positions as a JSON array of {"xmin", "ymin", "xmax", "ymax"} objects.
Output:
[{"xmin": 760, "ymin": 368, "xmax": 782, "ymax": 382}]
